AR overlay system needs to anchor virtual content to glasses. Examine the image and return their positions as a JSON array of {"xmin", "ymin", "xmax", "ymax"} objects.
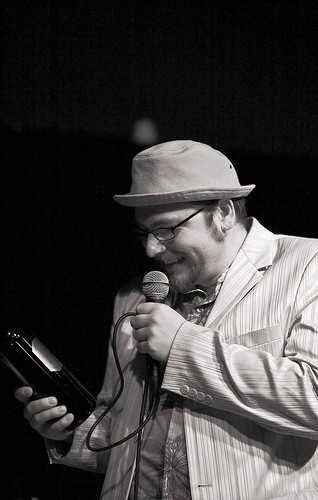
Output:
[{"xmin": 133, "ymin": 201, "xmax": 216, "ymax": 242}]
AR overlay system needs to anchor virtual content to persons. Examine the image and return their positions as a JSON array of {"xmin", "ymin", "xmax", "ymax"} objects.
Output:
[{"xmin": 15, "ymin": 140, "xmax": 318, "ymax": 500}]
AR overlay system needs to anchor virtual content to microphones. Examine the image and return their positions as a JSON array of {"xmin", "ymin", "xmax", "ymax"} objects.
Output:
[{"xmin": 142, "ymin": 271, "xmax": 170, "ymax": 400}]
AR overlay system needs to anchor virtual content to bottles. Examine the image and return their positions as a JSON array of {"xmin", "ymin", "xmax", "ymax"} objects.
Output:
[{"xmin": 0, "ymin": 326, "xmax": 98, "ymax": 430}]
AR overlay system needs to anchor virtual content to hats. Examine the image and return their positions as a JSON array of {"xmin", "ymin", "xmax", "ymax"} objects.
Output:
[{"xmin": 113, "ymin": 140, "xmax": 256, "ymax": 208}]
